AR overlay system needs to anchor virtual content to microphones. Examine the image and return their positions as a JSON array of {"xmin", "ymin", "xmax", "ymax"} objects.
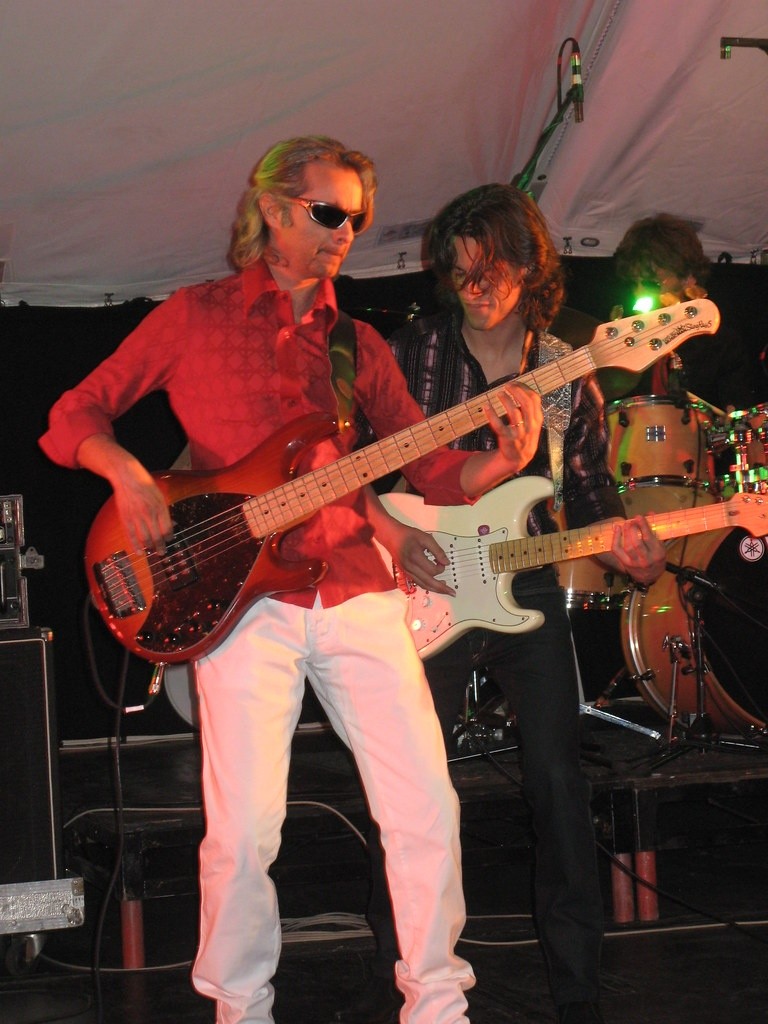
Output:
[
  {"xmin": 664, "ymin": 561, "xmax": 719, "ymax": 589},
  {"xmin": 571, "ymin": 41, "xmax": 584, "ymax": 123}
]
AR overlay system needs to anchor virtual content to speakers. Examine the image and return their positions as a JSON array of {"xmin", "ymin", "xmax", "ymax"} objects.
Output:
[{"xmin": 0, "ymin": 626, "xmax": 65, "ymax": 884}]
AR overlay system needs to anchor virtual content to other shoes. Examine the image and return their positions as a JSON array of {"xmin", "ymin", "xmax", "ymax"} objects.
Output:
[
  {"xmin": 556, "ymin": 1000, "xmax": 605, "ymax": 1024},
  {"xmin": 333, "ymin": 976, "xmax": 405, "ymax": 1024},
  {"xmin": 481, "ymin": 702, "xmax": 511, "ymax": 727}
]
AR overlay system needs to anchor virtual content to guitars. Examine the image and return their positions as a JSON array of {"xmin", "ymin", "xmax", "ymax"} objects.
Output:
[
  {"xmin": 84, "ymin": 285, "xmax": 721, "ymax": 666},
  {"xmin": 371, "ymin": 476, "xmax": 768, "ymax": 661}
]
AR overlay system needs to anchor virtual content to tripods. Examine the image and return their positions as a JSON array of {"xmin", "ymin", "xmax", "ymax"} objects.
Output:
[{"xmin": 637, "ymin": 580, "xmax": 768, "ymax": 777}]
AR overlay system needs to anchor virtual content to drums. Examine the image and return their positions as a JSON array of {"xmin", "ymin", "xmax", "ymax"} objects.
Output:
[{"xmin": 546, "ymin": 394, "xmax": 768, "ymax": 743}]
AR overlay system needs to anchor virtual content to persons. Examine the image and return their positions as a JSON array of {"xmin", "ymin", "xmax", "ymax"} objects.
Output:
[
  {"xmin": 37, "ymin": 137, "xmax": 543, "ymax": 1024},
  {"xmin": 352, "ymin": 181, "xmax": 666, "ymax": 1022},
  {"xmin": 581, "ymin": 210, "xmax": 712, "ymax": 403}
]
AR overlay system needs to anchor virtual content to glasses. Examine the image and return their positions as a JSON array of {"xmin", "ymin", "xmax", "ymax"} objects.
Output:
[{"xmin": 275, "ymin": 194, "xmax": 367, "ymax": 234}]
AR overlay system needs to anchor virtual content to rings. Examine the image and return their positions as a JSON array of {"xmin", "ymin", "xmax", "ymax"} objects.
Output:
[{"xmin": 510, "ymin": 420, "xmax": 525, "ymax": 428}]
